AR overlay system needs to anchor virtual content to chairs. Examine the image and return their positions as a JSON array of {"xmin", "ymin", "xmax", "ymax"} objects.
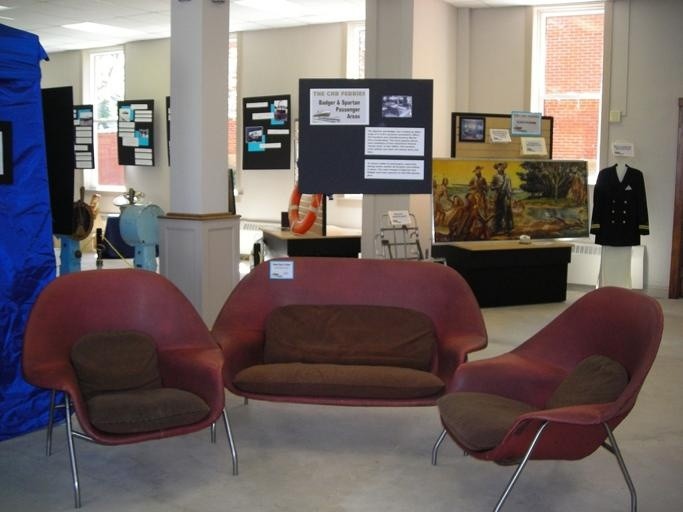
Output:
[
  {"xmin": 433, "ymin": 287, "xmax": 665, "ymax": 512},
  {"xmin": 23, "ymin": 268, "xmax": 239, "ymax": 511}
]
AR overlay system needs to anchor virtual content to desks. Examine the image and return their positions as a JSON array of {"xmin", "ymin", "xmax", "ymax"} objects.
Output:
[
  {"xmin": 432, "ymin": 239, "xmax": 575, "ymax": 308},
  {"xmin": 260, "ymin": 226, "xmax": 362, "ymax": 267}
]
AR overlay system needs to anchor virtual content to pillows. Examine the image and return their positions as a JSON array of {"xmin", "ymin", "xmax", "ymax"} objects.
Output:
[
  {"xmin": 262, "ymin": 304, "xmax": 434, "ymax": 370},
  {"xmin": 71, "ymin": 330, "xmax": 161, "ymax": 390},
  {"xmin": 87, "ymin": 388, "xmax": 209, "ymax": 437},
  {"xmin": 236, "ymin": 361, "xmax": 447, "ymax": 399},
  {"xmin": 440, "ymin": 394, "xmax": 540, "ymax": 454},
  {"xmin": 545, "ymin": 354, "xmax": 629, "ymax": 407}
]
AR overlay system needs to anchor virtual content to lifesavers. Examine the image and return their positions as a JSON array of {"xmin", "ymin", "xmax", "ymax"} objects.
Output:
[{"xmin": 288, "ymin": 181, "xmax": 322, "ymax": 234}]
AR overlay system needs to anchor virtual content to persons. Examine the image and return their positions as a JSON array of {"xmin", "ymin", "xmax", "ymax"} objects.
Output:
[{"xmin": 432, "ymin": 161, "xmax": 515, "ymax": 242}]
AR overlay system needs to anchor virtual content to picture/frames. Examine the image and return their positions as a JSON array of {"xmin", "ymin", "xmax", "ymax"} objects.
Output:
[
  {"xmin": 510, "ymin": 110, "xmax": 541, "ymax": 136},
  {"xmin": 458, "ymin": 116, "xmax": 485, "ymax": 143}
]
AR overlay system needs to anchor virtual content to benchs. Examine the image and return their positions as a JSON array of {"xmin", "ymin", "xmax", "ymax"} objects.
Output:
[{"xmin": 211, "ymin": 258, "xmax": 488, "ymax": 442}]
